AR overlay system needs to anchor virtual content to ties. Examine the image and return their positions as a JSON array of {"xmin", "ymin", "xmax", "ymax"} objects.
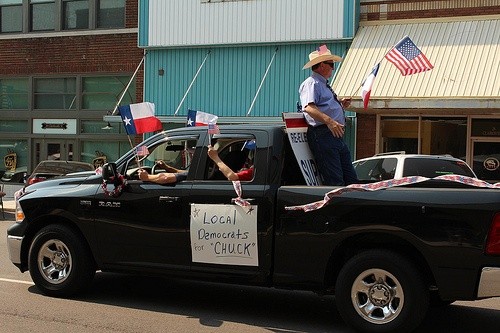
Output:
[{"xmin": 326, "ymin": 80, "xmax": 345, "ymax": 117}]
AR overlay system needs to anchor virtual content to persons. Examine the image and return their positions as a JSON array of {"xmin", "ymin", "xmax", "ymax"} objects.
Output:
[
  {"xmin": 298, "ymin": 44, "xmax": 359, "ymax": 187},
  {"xmin": 207, "ymin": 139, "xmax": 255, "ymax": 181},
  {"xmin": 137, "ymin": 141, "xmax": 196, "ymax": 185}
]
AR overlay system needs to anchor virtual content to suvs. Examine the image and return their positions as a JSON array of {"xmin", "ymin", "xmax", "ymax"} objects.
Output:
[{"xmin": 352, "ymin": 150, "xmax": 479, "ymax": 183}]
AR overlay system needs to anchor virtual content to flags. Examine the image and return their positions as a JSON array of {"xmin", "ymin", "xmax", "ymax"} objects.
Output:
[
  {"xmin": 187, "ymin": 109, "xmax": 218, "ymax": 127},
  {"xmin": 384, "ymin": 37, "xmax": 434, "ymax": 76},
  {"xmin": 117, "ymin": 101, "xmax": 163, "ymax": 135},
  {"xmin": 209, "ymin": 124, "xmax": 220, "ymax": 135},
  {"xmin": 137, "ymin": 146, "xmax": 149, "ymax": 156},
  {"xmin": 360, "ymin": 64, "xmax": 384, "ymax": 111}
]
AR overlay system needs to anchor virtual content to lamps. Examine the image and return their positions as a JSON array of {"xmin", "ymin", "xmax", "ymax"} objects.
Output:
[{"xmin": 101, "ymin": 110, "xmax": 113, "ymax": 130}]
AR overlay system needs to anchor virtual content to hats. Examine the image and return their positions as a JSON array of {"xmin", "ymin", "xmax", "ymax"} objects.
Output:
[
  {"xmin": 301, "ymin": 45, "xmax": 343, "ymax": 71},
  {"xmin": 245, "ymin": 140, "xmax": 255, "ymax": 149}
]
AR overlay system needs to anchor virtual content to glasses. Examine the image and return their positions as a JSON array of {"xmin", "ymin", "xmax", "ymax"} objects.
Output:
[{"xmin": 324, "ymin": 63, "xmax": 334, "ymax": 68}]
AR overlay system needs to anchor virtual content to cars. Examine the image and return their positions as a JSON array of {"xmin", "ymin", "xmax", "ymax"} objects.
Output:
[
  {"xmin": 1, "ymin": 166, "xmax": 29, "ymax": 184},
  {"xmin": 23, "ymin": 160, "xmax": 96, "ymax": 186}
]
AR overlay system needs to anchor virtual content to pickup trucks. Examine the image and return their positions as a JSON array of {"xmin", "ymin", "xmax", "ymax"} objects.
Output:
[{"xmin": 5, "ymin": 122, "xmax": 500, "ymax": 333}]
